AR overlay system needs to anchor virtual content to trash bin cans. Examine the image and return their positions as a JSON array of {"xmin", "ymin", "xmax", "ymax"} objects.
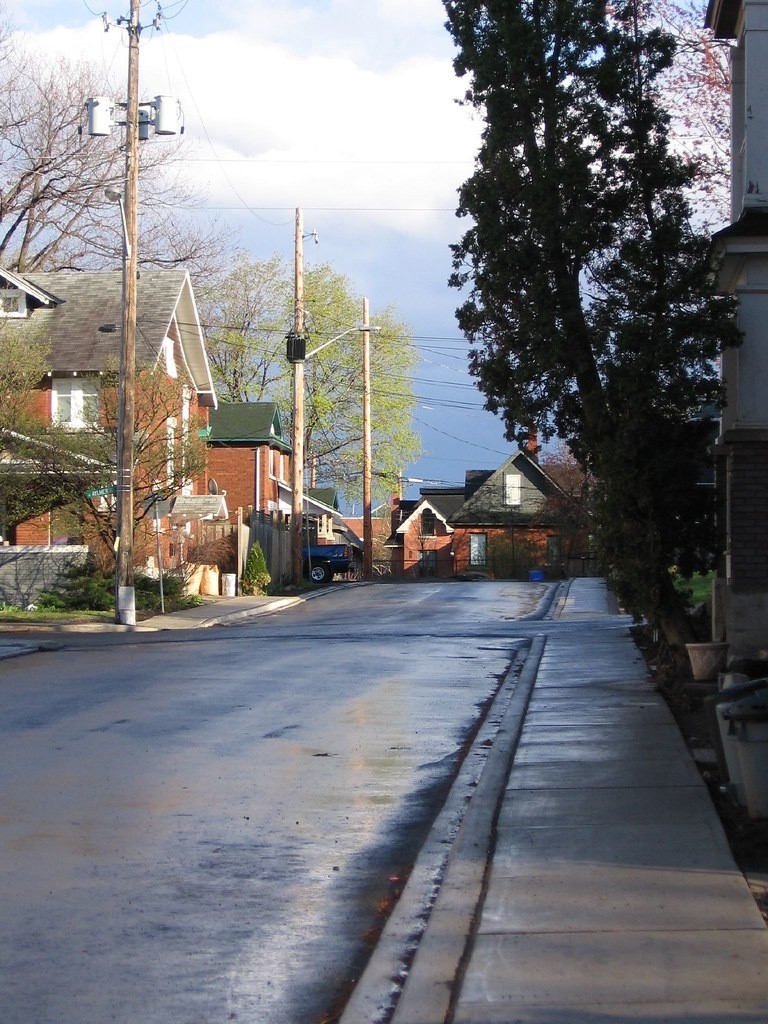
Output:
[
  {"xmin": 221, "ymin": 572, "xmax": 236, "ymax": 596},
  {"xmin": 705, "ymin": 676, "xmax": 768, "ymax": 821}
]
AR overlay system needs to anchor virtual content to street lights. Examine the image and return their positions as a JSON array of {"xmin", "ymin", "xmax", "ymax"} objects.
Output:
[
  {"xmin": 290, "ymin": 325, "xmax": 383, "ymax": 585},
  {"xmin": 101, "ymin": 184, "xmax": 140, "ymax": 626}
]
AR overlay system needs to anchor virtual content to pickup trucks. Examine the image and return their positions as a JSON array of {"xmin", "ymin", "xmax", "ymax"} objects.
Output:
[{"xmin": 302, "ymin": 543, "xmax": 355, "ymax": 585}]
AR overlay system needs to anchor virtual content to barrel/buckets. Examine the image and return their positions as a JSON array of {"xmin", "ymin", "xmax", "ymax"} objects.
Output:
[{"xmin": 221, "ymin": 573, "xmax": 236, "ymax": 598}]
